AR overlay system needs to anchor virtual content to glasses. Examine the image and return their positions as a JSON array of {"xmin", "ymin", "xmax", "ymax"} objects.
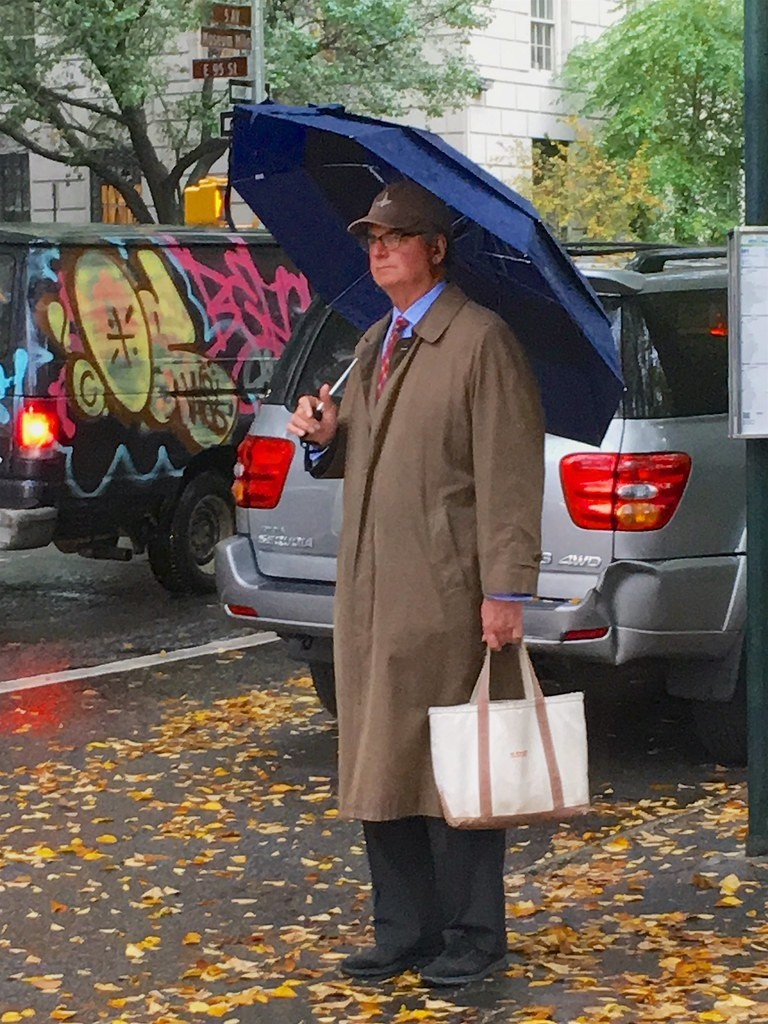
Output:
[{"xmin": 358, "ymin": 230, "xmax": 427, "ymax": 254}]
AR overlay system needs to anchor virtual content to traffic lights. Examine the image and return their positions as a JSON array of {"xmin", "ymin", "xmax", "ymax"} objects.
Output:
[{"xmin": 180, "ymin": 181, "xmax": 227, "ymax": 226}]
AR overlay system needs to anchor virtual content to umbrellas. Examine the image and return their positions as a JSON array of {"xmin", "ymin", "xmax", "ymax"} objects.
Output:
[{"xmin": 228, "ymin": 104, "xmax": 627, "ymax": 448}]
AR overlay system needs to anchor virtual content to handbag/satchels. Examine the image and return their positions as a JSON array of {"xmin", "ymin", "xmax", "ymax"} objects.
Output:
[{"xmin": 427, "ymin": 638, "xmax": 590, "ymax": 830}]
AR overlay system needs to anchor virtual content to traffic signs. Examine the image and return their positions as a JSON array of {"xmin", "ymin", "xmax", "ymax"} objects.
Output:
[
  {"xmin": 219, "ymin": 112, "xmax": 235, "ymax": 137},
  {"xmin": 230, "ymin": 79, "xmax": 271, "ymax": 106}
]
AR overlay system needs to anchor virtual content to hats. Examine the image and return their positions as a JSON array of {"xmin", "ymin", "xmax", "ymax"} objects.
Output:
[{"xmin": 347, "ymin": 182, "xmax": 455, "ymax": 254}]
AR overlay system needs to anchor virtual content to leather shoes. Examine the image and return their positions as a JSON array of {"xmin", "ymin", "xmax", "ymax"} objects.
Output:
[
  {"xmin": 340, "ymin": 937, "xmax": 445, "ymax": 974},
  {"xmin": 422, "ymin": 937, "xmax": 508, "ymax": 986}
]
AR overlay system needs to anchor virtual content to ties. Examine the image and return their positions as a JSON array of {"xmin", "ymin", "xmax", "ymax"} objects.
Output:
[{"xmin": 375, "ymin": 316, "xmax": 411, "ymax": 408}]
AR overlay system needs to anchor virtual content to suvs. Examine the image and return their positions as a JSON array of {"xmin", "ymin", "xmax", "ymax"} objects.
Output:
[
  {"xmin": 1, "ymin": 217, "xmax": 317, "ymax": 600},
  {"xmin": 210, "ymin": 238, "xmax": 745, "ymax": 769}
]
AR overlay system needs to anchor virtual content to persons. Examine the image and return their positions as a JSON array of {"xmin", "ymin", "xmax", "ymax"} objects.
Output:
[{"xmin": 287, "ymin": 180, "xmax": 545, "ymax": 987}]
